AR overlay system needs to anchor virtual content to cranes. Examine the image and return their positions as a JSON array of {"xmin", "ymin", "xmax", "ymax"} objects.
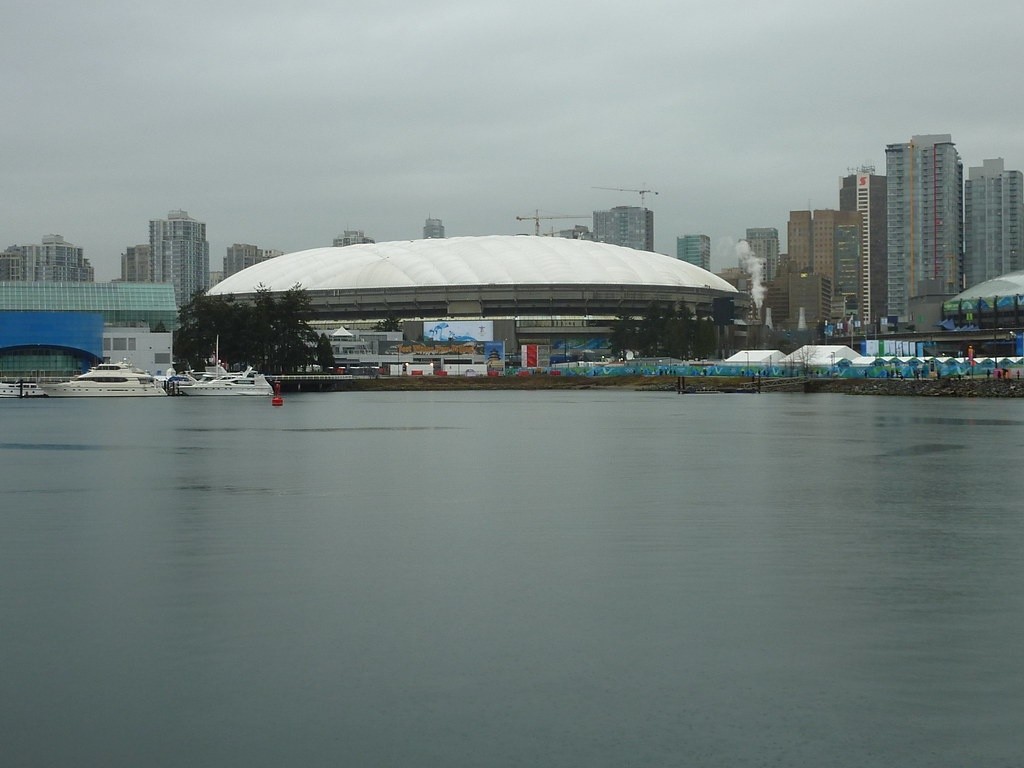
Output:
[
  {"xmin": 591, "ymin": 186, "xmax": 658, "ymax": 208},
  {"xmin": 515, "ymin": 209, "xmax": 592, "ymax": 236}
]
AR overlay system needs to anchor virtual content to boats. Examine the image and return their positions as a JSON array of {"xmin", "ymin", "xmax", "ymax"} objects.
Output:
[
  {"xmin": 36, "ymin": 357, "xmax": 168, "ymax": 398},
  {"xmin": 152, "ymin": 330, "xmax": 275, "ymax": 397},
  {"xmin": 0, "ymin": 382, "xmax": 45, "ymax": 398}
]
